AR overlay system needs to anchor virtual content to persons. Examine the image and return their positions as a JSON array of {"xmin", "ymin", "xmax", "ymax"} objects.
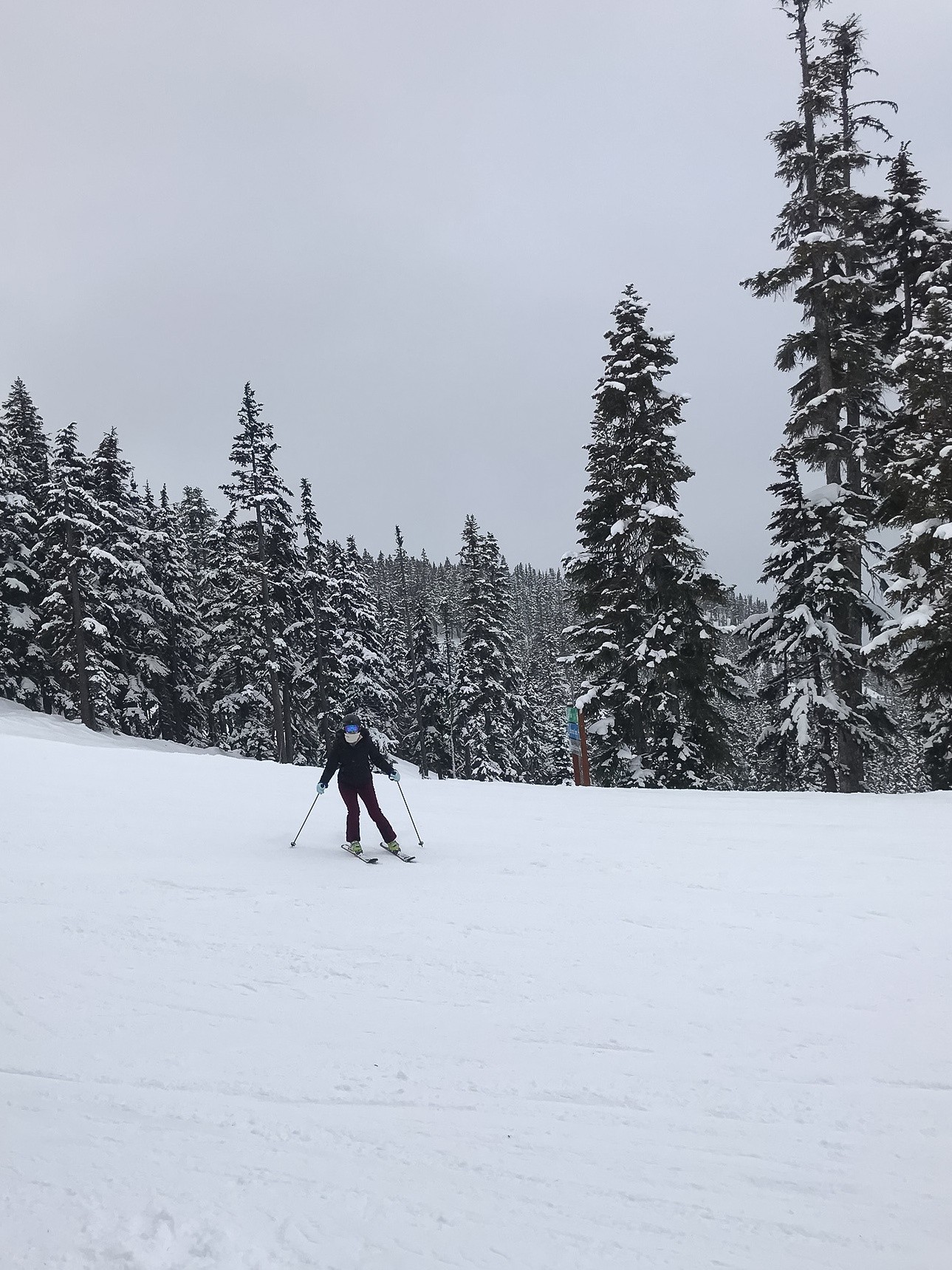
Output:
[{"xmin": 315, "ymin": 712, "xmax": 401, "ymax": 855}]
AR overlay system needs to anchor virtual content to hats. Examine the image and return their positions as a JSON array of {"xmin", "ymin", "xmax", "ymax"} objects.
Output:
[{"xmin": 342, "ymin": 714, "xmax": 361, "ymax": 726}]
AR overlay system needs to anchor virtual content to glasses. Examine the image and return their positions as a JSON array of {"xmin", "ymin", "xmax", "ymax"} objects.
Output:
[{"xmin": 343, "ymin": 725, "xmax": 360, "ymax": 733}]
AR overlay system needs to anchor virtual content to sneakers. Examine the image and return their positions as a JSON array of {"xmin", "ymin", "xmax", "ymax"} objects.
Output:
[
  {"xmin": 350, "ymin": 842, "xmax": 363, "ymax": 855},
  {"xmin": 388, "ymin": 840, "xmax": 401, "ymax": 855}
]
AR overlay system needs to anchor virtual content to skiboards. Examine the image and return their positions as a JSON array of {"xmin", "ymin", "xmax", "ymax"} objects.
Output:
[{"xmin": 340, "ymin": 841, "xmax": 416, "ymax": 864}]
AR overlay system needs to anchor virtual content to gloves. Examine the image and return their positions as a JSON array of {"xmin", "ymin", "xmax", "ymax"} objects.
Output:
[
  {"xmin": 316, "ymin": 782, "xmax": 326, "ymax": 793},
  {"xmin": 389, "ymin": 769, "xmax": 400, "ymax": 782}
]
